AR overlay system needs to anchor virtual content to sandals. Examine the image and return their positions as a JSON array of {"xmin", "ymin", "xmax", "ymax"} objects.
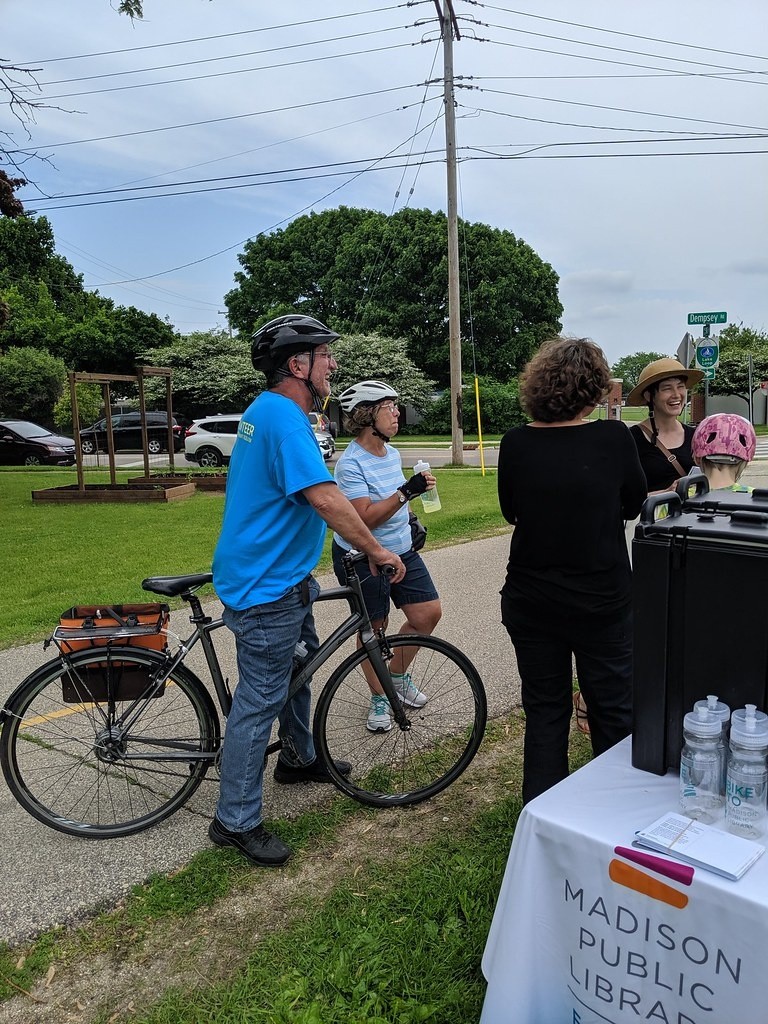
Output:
[{"xmin": 573, "ymin": 691, "xmax": 590, "ymax": 734}]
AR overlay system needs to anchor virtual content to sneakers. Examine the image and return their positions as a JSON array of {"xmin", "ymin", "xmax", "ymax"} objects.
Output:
[
  {"xmin": 208, "ymin": 814, "xmax": 291, "ymax": 868},
  {"xmin": 366, "ymin": 693, "xmax": 392, "ymax": 732},
  {"xmin": 387, "ymin": 666, "xmax": 427, "ymax": 707},
  {"xmin": 274, "ymin": 757, "xmax": 352, "ymax": 784}
]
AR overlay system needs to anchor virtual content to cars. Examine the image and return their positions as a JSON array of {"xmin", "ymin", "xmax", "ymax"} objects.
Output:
[{"xmin": 0, "ymin": 418, "xmax": 77, "ymax": 467}]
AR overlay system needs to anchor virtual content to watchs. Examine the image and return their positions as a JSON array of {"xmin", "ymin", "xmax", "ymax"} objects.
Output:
[{"xmin": 396, "ymin": 490, "xmax": 407, "ymax": 504}]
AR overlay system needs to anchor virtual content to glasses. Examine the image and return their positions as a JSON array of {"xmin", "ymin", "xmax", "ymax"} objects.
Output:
[{"xmin": 381, "ymin": 404, "xmax": 397, "ymax": 413}]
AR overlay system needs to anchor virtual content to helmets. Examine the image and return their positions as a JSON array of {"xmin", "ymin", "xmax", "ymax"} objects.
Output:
[
  {"xmin": 249, "ymin": 315, "xmax": 340, "ymax": 371},
  {"xmin": 691, "ymin": 413, "xmax": 757, "ymax": 464},
  {"xmin": 338, "ymin": 380, "xmax": 398, "ymax": 418}
]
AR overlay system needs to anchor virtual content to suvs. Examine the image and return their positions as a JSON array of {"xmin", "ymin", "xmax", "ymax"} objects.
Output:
[
  {"xmin": 307, "ymin": 411, "xmax": 337, "ymax": 440},
  {"xmin": 184, "ymin": 410, "xmax": 336, "ymax": 467}
]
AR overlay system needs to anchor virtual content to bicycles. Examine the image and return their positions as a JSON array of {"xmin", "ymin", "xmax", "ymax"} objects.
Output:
[{"xmin": 0, "ymin": 548, "xmax": 489, "ymax": 840}]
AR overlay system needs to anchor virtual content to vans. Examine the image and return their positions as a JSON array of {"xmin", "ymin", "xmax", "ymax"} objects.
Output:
[{"xmin": 79, "ymin": 409, "xmax": 190, "ymax": 455}]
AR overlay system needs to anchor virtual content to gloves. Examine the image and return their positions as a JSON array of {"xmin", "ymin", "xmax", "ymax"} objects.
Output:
[{"xmin": 408, "ymin": 512, "xmax": 427, "ymax": 553}]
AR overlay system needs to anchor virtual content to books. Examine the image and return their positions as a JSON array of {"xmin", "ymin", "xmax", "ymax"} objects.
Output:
[{"xmin": 636, "ymin": 810, "xmax": 766, "ymax": 881}]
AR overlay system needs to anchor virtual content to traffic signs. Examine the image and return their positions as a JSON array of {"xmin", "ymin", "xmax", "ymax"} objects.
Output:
[{"xmin": 694, "ymin": 336, "xmax": 720, "ymax": 381}]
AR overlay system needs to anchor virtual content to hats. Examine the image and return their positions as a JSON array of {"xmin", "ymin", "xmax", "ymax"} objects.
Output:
[{"xmin": 626, "ymin": 358, "xmax": 705, "ymax": 407}]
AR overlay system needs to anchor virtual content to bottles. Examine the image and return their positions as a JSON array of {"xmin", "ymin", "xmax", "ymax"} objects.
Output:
[
  {"xmin": 295, "ymin": 640, "xmax": 308, "ymax": 657},
  {"xmin": 678, "ymin": 695, "xmax": 767, "ymax": 839},
  {"xmin": 414, "ymin": 460, "xmax": 441, "ymax": 513}
]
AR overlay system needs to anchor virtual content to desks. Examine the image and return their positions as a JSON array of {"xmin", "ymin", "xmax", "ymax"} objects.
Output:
[{"xmin": 479, "ymin": 727, "xmax": 768, "ymax": 1024}]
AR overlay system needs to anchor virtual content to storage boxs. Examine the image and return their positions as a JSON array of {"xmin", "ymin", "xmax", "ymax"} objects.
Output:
[{"xmin": 58, "ymin": 600, "xmax": 169, "ymax": 702}]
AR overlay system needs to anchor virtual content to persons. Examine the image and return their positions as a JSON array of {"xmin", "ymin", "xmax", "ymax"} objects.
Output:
[
  {"xmin": 574, "ymin": 357, "xmax": 700, "ymax": 734},
  {"xmin": 206, "ymin": 314, "xmax": 407, "ymax": 867},
  {"xmin": 654, "ymin": 412, "xmax": 758, "ymax": 524},
  {"xmin": 496, "ymin": 338, "xmax": 647, "ymax": 811},
  {"xmin": 331, "ymin": 380, "xmax": 443, "ymax": 733}
]
